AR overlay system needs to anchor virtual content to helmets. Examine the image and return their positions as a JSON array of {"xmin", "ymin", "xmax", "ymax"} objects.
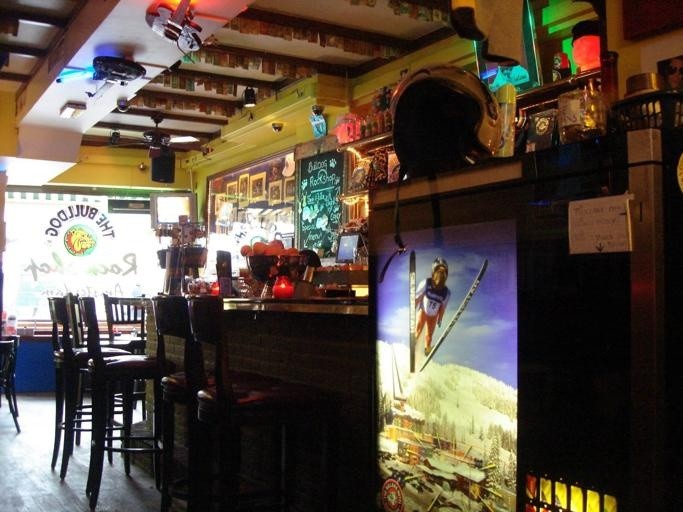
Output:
[
  {"xmin": 389, "ymin": 64, "xmax": 502, "ymax": 177},
  {"xmin": 431, "ymin": 256, "xmax": 448, "ymax": 278}
]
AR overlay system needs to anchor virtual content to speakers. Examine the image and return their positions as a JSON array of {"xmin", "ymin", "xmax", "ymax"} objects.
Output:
[{"xmin": 152, "ymin": 151, "xmax": 176, "ymax": 183}]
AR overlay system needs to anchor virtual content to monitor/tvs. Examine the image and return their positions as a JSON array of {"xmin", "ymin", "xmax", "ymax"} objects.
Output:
[
  {"xmin": 150, "ymin": 192, "xmax": 198, "ymax": 229},
  {"xmin": 335, "ymin": 231, "xmax": 361, "ymax": 263}
]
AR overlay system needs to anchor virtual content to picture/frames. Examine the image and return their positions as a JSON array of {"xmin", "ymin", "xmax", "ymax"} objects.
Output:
[{"xmin": 202, "ymin": 144, "xmax": 299, "ymax": 276}]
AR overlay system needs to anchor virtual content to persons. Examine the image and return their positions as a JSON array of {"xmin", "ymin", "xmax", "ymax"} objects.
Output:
[{"xmin": 414, "ymin": 257, "xmax": 450, "ymax": 356}]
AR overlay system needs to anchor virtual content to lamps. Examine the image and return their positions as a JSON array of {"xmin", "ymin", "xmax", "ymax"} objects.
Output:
[
  {"xmin": 142, "ymin": 113, "xmax": 170, "ymax": 150},
  {"xmin": 242, "ymin": 81, "xmax": 255, "ymax": 108}
]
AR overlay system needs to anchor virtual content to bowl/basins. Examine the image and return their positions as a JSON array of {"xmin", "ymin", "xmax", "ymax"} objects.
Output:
[{"xmin": 246, "ymin": 255, "xmax": 311, "ymax": 287}]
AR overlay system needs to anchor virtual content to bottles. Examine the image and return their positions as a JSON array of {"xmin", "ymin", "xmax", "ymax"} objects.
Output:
[
  {"xmin": 495, "ymin": 83, "xmax": 517, "ymax": 158},
  {"xmin": 359, "ymin": 110, "xmax": 393, "ymax": 140},
  {"xmin": 6, "ymin": 316, "xmax": 17, "ymax": 336}
]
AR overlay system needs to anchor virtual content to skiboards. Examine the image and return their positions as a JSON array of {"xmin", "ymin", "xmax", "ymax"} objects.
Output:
[{"xmin": 409, "ymin": 250, "xmax": 489, "ymax": 374}]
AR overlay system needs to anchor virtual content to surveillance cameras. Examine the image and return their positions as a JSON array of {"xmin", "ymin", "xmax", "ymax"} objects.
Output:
[
  {"xmin": 139, "ymin": 164, "xmax": 146, "ymax": 170},
  {"xmin": 312, "ymin": 105, "xmax": 325, "ymax": 116},
  {"xmin": 272, "ymin": 123, "xmax": 284, "ymax": 134},
  {"xmin": 117, "ymin": 100, "xmax": 129, "ymax": 113}
]
